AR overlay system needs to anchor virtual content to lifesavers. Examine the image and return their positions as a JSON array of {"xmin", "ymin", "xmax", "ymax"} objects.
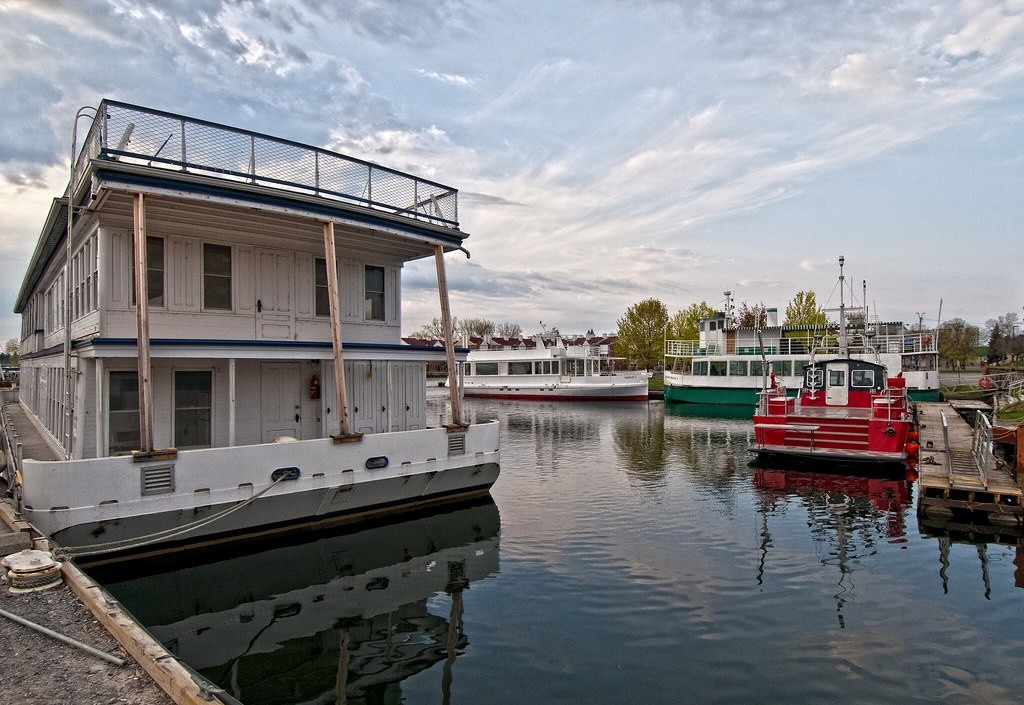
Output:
[{"xmin": 978, "ymin": 377, "xmax": 991, "ymax": 387}]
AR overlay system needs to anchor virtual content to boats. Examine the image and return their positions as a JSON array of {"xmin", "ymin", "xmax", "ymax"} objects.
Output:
[
  {"xmin": 443, "ymin": 346, "xmax": 651, "ymax": 400},
  {"xmin": 752, "ymin": 255, "xmax": 920, "ymax": 471},
  {"xmin": 659, "ymin": 284, "xmax": 942, "ymax": 403}
]
